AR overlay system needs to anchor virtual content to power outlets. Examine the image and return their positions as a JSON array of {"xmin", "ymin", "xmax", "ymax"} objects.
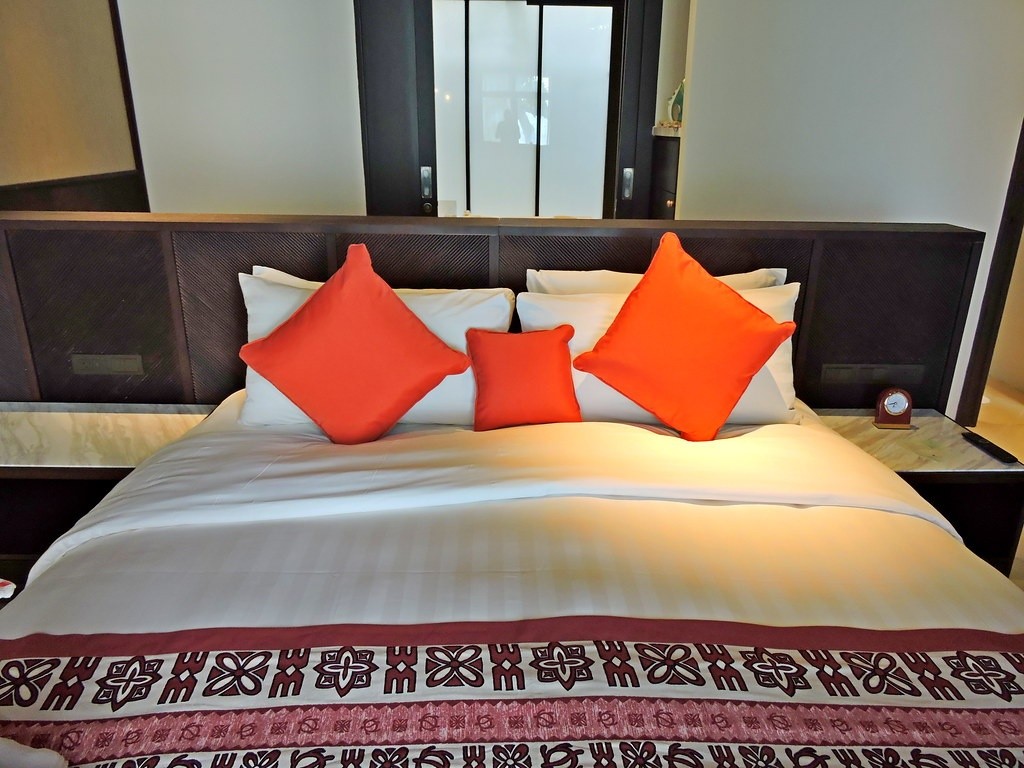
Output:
[
  {"xmin": 890, "ymin": 365, "xmax": 925, "ymax": 385},
  {"xmin": 70, "ymin": 354, "xmax": 103, "ymax": 375}
]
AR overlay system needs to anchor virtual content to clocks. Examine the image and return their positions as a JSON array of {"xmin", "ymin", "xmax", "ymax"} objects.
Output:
[{"xmin": 872, "ymin": 388, "xmax": 912, "ymax": 430}]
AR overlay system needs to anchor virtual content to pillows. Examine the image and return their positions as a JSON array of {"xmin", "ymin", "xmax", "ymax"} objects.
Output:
[
  {"xmin": 238, "ymin": 243, "xmax": 475, "ymax": 445},
  {"xmin": 238, "ymin": 266, "xmax": 514, "ymax": 426},
  {"xmin": 465, "ymin": 325, "xmax": 584, "ymax": 431},
  {"xmin": 572, "ymin": 231, "xmax": 799, "ymax": 442},
  {"xmin": 516, "ymin": 269, "xmax": 805, "ymax": 427}
]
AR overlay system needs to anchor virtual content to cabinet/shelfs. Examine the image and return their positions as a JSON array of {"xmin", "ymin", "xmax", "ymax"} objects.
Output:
[{"xmin": 649, "ymin": 136, "xmax": 681, "ymax": 220}]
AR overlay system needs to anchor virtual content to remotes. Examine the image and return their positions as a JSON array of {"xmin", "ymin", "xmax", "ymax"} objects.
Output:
[{"xmin": 961, "ymin": 432, "xmax": 1018, "ymax": 464}]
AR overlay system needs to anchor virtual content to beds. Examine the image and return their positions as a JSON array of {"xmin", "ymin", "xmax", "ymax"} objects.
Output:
[{"xmin": 0, "ymin": 386, "xmax": 1024, "ymax": 768}]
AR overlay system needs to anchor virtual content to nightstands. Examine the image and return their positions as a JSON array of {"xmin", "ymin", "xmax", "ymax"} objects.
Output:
[{"xmin": 814, "ymin": 408, "xmax": 1024, "ymax": 578}]
